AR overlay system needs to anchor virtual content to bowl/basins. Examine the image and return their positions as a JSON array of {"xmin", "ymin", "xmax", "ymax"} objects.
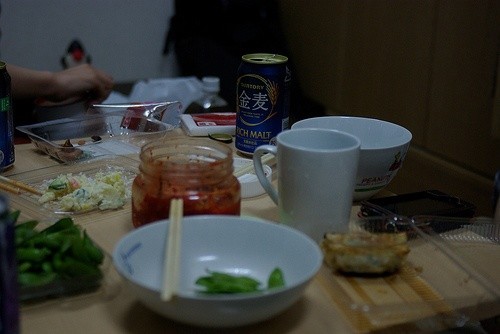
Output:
[
  {"xmin": 290, "ymin": 117, "xmax": 412, "ymax": 201},
  {"xmin": 316, "ymin": 213, "xmax": 500, "ymax": 334},
  {"xmin": 111, "ymin": 214, "xmax": 323, "ymax": 329},
  {"xmin": 361, "ymin": 190, "xmax": 473, "ymax": 224},
  {"xmin": 16, "ymin": 110, "xmax": 175, "ymax": 163}
]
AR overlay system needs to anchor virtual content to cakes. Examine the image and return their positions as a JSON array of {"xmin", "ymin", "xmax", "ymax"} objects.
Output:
[{"xmin": 40, "ymin": 171, "xmax": 125, "ymax": 213}]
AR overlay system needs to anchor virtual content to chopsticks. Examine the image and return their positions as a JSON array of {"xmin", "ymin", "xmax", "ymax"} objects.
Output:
[
  {"xmin": 0, "ymin": 174, "xmax": 45, "ymax": 196},
  {"xmin": 162, "ymin": 198, "xmax": 184, "ymax": 302},
  {"xmin": 232, "ymin": 153, "xmax": 277, "ymax": 179}
]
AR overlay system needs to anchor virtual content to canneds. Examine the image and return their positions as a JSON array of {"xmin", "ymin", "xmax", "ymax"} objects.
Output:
[
  {"xmin": 235, "ymin": 53, "xmax": 290, "ymax": 156},
  {"xmin": 132, "ymin": 136, "xmax": 242, "ymax": 225},
  {"xmin": 0, "ymin": 61, "xmax": 16, "ymax": 172}
]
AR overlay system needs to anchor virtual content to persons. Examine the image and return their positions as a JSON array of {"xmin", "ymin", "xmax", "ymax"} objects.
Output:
[{"xmin": 3, "ymin": 63, "xmax": 113, "ymax": 108}]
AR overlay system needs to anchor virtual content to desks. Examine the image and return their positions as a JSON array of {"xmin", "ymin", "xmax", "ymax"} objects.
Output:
[{"xmin": 0, "ymin": 128, "xmax": 499, "ymax": 334}]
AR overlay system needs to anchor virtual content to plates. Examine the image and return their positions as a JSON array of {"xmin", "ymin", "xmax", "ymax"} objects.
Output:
[
  {"xmin": 0, "ymin": 155, "xmax": 142, "ymax": 221},
  {"xmin": 0, "ymin": 222, "xmax": 127, "ymax": 311}
]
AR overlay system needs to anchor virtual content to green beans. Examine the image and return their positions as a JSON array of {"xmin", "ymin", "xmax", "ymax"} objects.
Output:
[
  {"xmin": 196, "ymin": 267, "xmax": 285, "ymax": 293},
  {"xmin": 10, "ymin": 207, "xmax": 106, "ymax": 292}
]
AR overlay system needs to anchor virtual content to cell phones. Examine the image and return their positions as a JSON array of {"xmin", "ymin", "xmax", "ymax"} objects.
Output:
[{"xmin": 360, "ymin": 189, "xmax": 478, "ymax": 233}]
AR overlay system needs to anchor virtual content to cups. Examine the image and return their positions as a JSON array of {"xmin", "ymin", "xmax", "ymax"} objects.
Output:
[{"xmin": 254, "ymin": 128, "xmax": 361, "ymax": 246}]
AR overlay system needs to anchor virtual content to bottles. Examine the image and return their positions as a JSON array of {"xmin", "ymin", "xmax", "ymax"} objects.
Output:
[
  {"xmin": 132, "ymin": 137, "xmax": 241, "ymax": 228},
  {"xmin": 184, "ymin": 77, "xmax": 228, "ymax": 114}
]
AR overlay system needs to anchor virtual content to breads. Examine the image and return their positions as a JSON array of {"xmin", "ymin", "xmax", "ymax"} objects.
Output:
[{"xmin": 322, "ymin": 230, "xmax": 408, "ymax": 275}]
